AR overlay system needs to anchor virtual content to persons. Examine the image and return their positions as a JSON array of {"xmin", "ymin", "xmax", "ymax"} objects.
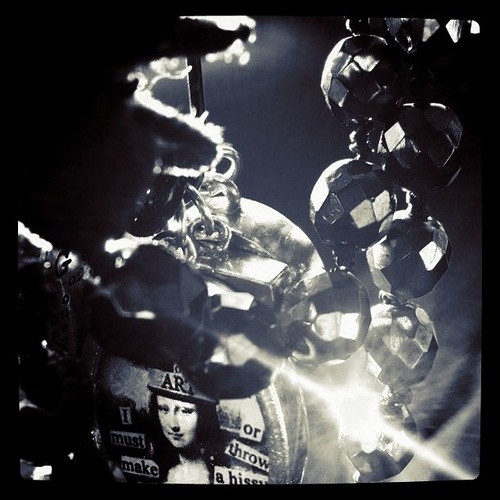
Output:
[{"xmin": 140, "ymin": 358, "xmax": 231, "ymax": 484}]
